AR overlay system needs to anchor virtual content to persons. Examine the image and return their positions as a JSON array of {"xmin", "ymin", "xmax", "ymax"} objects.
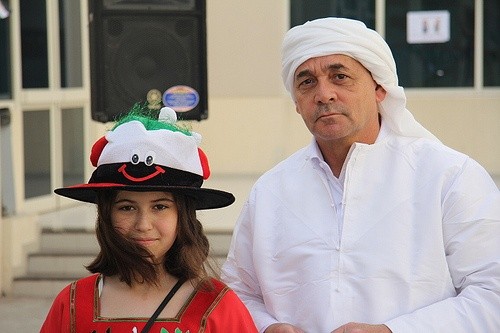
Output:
[
  {"xmin": 217, "ymin": 16, "xmax": 499, "ymax": 332},
  {"xmin": 38, "ymin": 99, "xmax": 258, "ymax": 332}
]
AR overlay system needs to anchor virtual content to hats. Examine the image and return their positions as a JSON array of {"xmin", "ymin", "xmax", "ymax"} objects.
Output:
[{"xmin": 54, "ymin": 106, "xmax": 235, "ymax": 210}]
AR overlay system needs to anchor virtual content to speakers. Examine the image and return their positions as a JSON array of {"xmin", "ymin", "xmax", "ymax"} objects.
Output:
[{"xmin": 88, "ymin": 0, "xmax": 211, "ymax": 122}]
